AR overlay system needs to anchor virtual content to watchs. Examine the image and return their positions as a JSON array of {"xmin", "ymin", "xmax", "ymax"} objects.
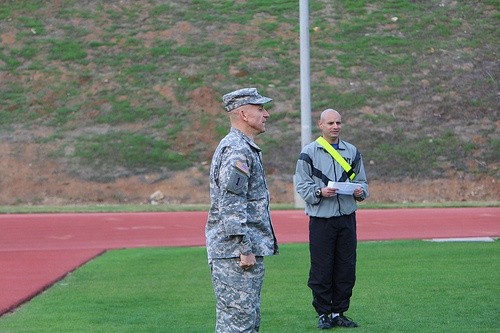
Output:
[{"xmin": 316, "ymin": 187, "xmax": 321, "ymax": 197}]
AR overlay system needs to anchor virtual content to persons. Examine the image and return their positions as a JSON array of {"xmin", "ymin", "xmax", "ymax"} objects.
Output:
[
  {"xmin": 206, "ymin": 88, "xmax": 279, "ymax": 333},
  {"xmin": 295, "ymin": 109, "xmax": 369, "ymax": 330}
]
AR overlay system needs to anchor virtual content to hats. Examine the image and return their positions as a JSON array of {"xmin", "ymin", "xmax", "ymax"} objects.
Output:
[{"xmin": 223, "ymin": 87, "xmax": 273, "ymax": 112}]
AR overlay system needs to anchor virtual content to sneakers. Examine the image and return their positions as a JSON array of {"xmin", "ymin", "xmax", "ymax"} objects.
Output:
[
  {"xmin": 331, "ymin": 313, "xmax": 358, "ymax": 327},
  {"xmin": 318, "ymin": 313, "xmax": 331, "ymax": 329}
]
